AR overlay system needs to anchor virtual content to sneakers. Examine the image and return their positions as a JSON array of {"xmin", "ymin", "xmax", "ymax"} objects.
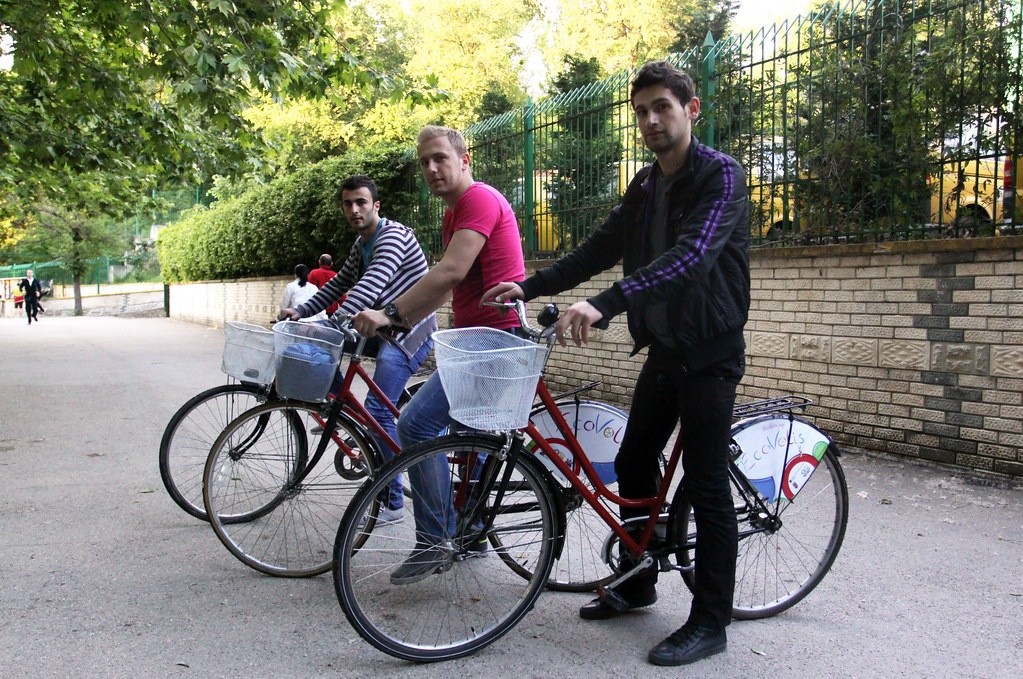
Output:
[
  {"xmin": 310, "ymin": 422, "xmax": 341, "ymax": 435},
  {"xmin": 357, "ymin": 506, "xmax": 405, "ymax": 529},
  {"xmin": 648, "ymin": 619, "xmax": 727, "ymax": 666},
  {"xmin": 580, "ymin": 588, "xmax": 657, "ymax": 620},
  {"xmin": 452, "ymin": 530, "xmax": 488, "ymax": 558},
  {"xmin": 390, "ymin": 541, "xmax": 454, "ymax": 585}
]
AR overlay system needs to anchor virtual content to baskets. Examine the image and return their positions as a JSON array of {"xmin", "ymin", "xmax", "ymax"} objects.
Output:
[
  {"xmin": 271, "ymin": 321, "xmax": 345, "ymax": 404},
  {"xmin": 431, "ymin": 327, "xmax": 548, "ymax": 431},
  {"xmin": 221, "ymin": 320, "xmax": 278, "ymax": 384}
]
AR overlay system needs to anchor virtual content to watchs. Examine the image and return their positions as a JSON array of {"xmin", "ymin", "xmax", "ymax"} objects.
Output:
[{"xmin": 384, "ymin": 302, "xmax": 402, "ymax": 322}]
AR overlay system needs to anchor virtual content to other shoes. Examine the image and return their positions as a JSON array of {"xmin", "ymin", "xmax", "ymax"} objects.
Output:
[{"xmin": 31, "ymin": 314, "xmax": 38, "ymax": 321}]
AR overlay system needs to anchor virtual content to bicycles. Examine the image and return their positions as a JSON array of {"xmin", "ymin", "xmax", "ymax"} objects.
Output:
[
  {"xmin": 157, "ymin": 297, "xmax": 672, "ymax": 594},
  {"xmin": 330, "ymin": 295, "xmax": 851, "ymax": 664}
]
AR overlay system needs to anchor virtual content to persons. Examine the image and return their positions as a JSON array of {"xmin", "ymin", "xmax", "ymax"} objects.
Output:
[
  {"xmin": 10, "ymin": 270, "xmax": 47, "ymax": 325},
  {"xmin": 277, "ymin": 175, "xmax": 438, "ymax": 530},
  {"xmin": 350, "ymin": 126, "xmax": 525, "ymax": 586},
  {"xmin": 479, "ymin": 61, "xmax": 752, "ymax": 667}
]
